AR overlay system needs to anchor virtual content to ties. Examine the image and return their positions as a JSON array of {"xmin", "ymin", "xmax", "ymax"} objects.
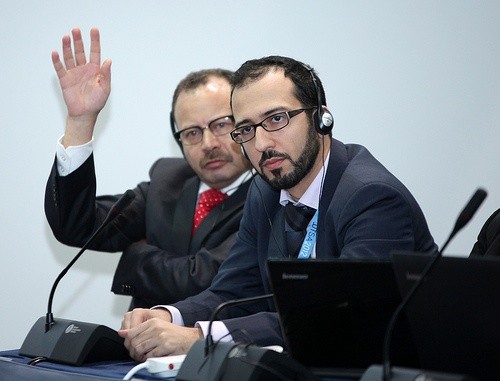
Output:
[{"xmin": 192, "ymin": 189, "xmax": 228, "ymax": 236}]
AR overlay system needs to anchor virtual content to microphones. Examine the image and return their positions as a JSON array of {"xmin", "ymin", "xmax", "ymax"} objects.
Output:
[
  {"xmin": 382, "ymin": 188, "xmax": 487, "ymax": 381},
  {"xmin": 20, "ymin": 190, "xmax": 138, "ymax": 366}
]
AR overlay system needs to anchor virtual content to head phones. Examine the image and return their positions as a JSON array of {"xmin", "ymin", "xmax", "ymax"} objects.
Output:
[{"xmin": 240, "ymin": 62, "xmax": 334, "ymax": 160}]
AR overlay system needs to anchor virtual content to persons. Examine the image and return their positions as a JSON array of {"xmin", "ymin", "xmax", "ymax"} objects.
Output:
[
  {"xmin": 44, "ymin": 26, "xmax": 257, "ymax": 312},
  {"xmin": 118, "ymin": 56, "xmax": 439, "ymax": 363}
]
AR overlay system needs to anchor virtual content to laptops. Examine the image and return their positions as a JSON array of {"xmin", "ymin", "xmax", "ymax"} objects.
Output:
[{"xmin": 265, "ymin": 253, "xmax": 500, "ymax": 378}]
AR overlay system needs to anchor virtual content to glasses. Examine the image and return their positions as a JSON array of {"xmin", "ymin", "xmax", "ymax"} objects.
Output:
[
  {"xmin": 174, "ymin": 115, "xmax": 236, "ymax": 145},
  {"xmin": 229, "ymin": 106, "xmax": 317, "ymax": 144}
]
